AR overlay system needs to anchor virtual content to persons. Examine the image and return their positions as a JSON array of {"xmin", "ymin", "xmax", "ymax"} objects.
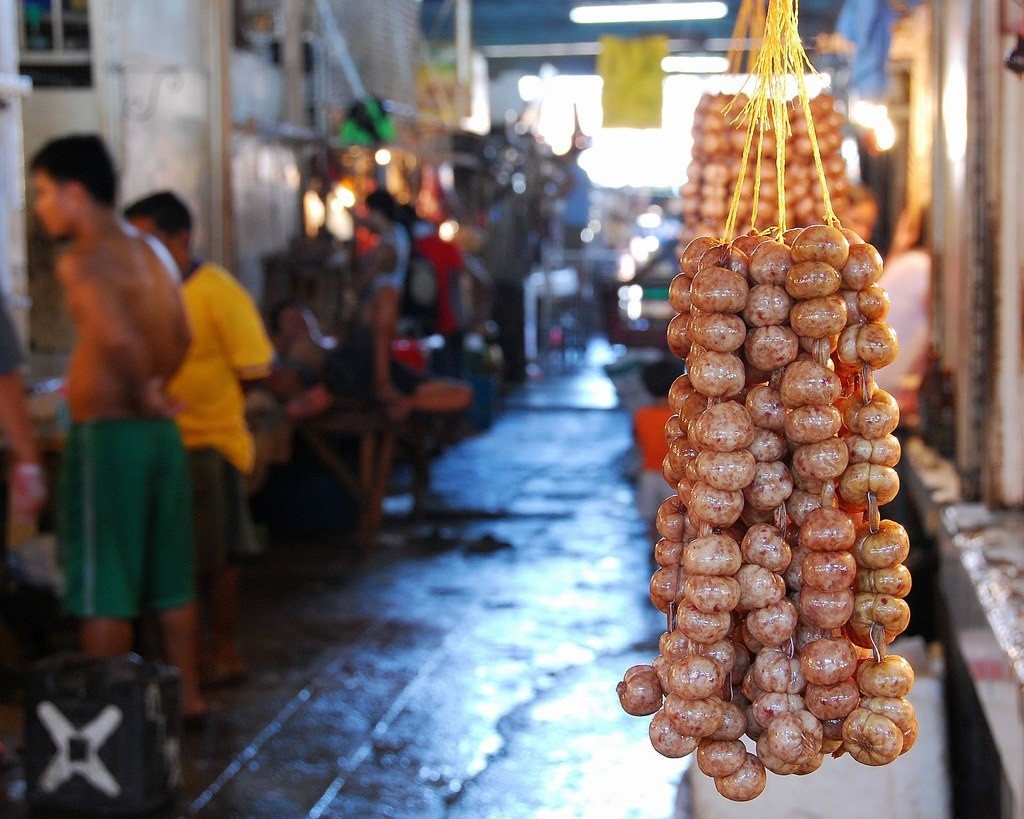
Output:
[
  {"xmin": 0, "ymin": 133, "xmax": 528, "ymax": 731},
  {"xmin": 846, "ymin": 186, "xmax": 932, "ymax": 425}
]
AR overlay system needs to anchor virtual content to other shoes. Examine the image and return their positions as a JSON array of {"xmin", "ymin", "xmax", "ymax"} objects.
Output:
[
  {"xmin": 201, "ymin": 650, "xmax": 245, "ymax": 686},
  {"xmin": 156, "ymin": 678, "xmax": 208, "ymax": 736}
]
